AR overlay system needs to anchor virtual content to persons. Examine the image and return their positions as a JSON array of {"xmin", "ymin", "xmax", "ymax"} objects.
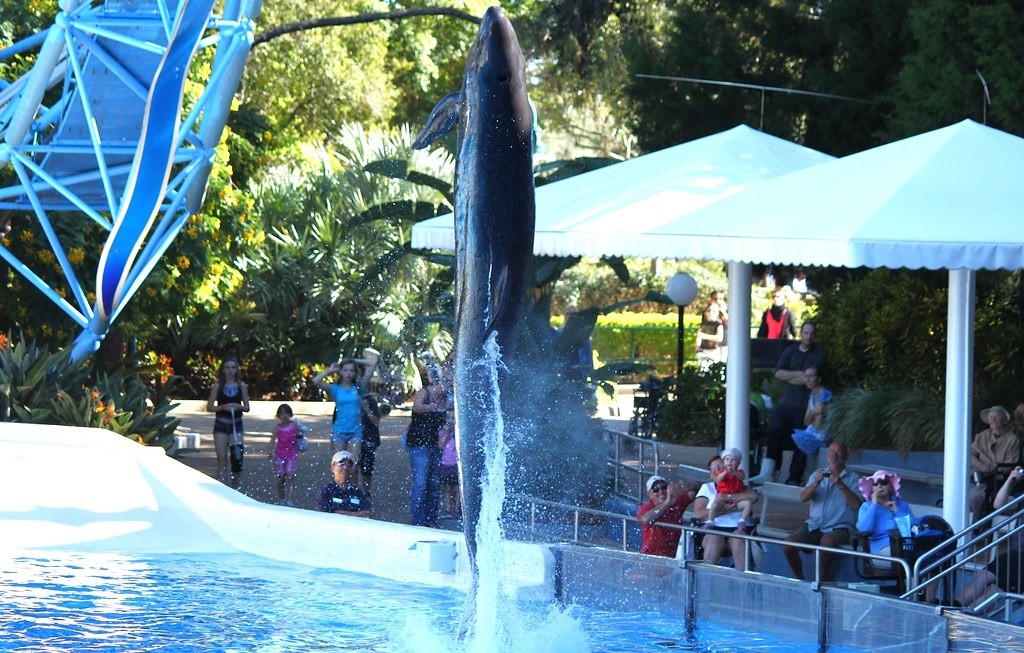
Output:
[
  {"xmin": 312, "ymin": 358, "xmax": 375, "ymax": 486},
  {"xmin": 268, "ymin": 404, "xmax": 303, "ymax": 508},
  {"xmin": 359, "ymin": 393, "xmax": 380, "ymax": 495},
  {"xmin": 785, "ymin": 365, "xmax": 834, "ymax": 487},
  {"xmin": 207, "ymin": 356, "xmax": 249, "ymax": 492},
  {"xmin": 856, "ymin": 470, "xmax": 939, "ymax": 604},
  {"xmin": 709, "ymin": 447, "xmax": 752, "ymax": 535},
  {"xmin": 637, "ymin": 476, "xmax": 704, "ymax": 558},
  {"xmin": 749, "ymin": 323, "xmax": 827, "ymax": 488},
  {"xmin": 696, "ymin": 303, "xmax": 724, "ymax": 371},
  {"xmin": 946, "ymin": 405, "xmax": 1024, "ymax": 617},
  {"xmin": 695, "ymin": 457, "xmax": 758, "ymax": 573},
  {"xmin": 783, "ymin": 440, "xmax": 867, "ymax": 586},
  {"xmin": 316, "ymin": 451, "xmax": 374, "ymax": 519},
  {"xmin": 406, "ymin": 374, "xmax": 454, "ymax": 528},
  {"xmin": 758, "ymin": 289, "xmax": 799, "ymax": 341},
  {"xmin": 438, "ymin": 409, "xmax": 461, "ymax": 519},
  {"xmin": 708, "ymin": 292, "xmax": 728, "ymax": 365}
]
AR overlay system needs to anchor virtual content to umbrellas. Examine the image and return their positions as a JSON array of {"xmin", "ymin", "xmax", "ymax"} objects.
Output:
[
  {"xmin": 636, "ymin": 117, "xmax": 1024, "ymax": 565},
  {"xmin": 415, "ymin": 125, "xmax": 840, "ymax": 482}
]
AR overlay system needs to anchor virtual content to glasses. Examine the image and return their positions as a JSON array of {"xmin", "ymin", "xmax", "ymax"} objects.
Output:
[
  {"xmin": 650, "ymin": 485, "xmax": 667, "ymax": 492},
  {"xmin": 872, "ymin": 479, "xmax": 889, "ymax": 486},
  {"xmin": 337, "ymin": 461, "xmax": 353, "ymax": 467}
]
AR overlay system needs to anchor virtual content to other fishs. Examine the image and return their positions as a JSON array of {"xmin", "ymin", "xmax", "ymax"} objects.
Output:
[{"xmin": 412, "ymin": 6, "xmax": 537, "ymax": 607}]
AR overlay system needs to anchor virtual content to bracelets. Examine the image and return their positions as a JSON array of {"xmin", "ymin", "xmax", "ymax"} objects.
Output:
[
  {"xmin": 728, "ymin": 494, "xmax": 732, "ymax": 501},
  {"xmin": 341, "ymin": 510, "xmax": 345, "ymax": 515}
]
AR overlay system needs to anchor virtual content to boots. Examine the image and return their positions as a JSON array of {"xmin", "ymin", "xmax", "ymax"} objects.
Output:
[{"xmin": 749, "ymin": 458, "xmax": 777, "ymax": 484}]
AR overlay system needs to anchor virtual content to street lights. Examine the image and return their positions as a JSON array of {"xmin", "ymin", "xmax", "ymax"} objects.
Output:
[{"xmin": 666, "ymin": 272, "xmax": 698, "ymax": 378}]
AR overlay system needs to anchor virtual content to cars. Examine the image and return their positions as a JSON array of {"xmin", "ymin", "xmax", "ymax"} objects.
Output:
[
  {"xmin": 751, "ymin": 338, "xmax": 796, "ymax": 415},
  {"xmin": 597, "ymin": 363, "xmax": 655, "ymax": 384}
]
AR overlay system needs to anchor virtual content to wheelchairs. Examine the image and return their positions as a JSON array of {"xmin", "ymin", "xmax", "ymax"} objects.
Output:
[{"xmin": 629, "ymin": 388, "xmax": 659, "ymax": 439}]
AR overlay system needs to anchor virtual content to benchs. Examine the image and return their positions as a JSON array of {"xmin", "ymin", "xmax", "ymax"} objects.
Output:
[{"xmin": 677, "ymin": 461, "xmax": 1020, "ymax": 574}]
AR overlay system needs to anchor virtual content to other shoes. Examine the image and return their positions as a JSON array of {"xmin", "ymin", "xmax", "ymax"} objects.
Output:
[
  {"xmin": 738, "ymin": 521, "xmax": 747, "ymax": 531},
  {"xmin": 701, "ymin": 522, "xmax": 714, "ymax": 529},
  {"xmin": 934, "ymin": 599, "xmax": 961, "ymax": 607}
]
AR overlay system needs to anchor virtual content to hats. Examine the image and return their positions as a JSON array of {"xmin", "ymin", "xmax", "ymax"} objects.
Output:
[
  {"xmin": 331, "ymin": 451, "xmax": 355, "ymax": 465},
  {"xmin": 646, "ymin": 475, "xmax": 666, "ymax": 492},
  {"xmin": 980, "ymin": 406, "xmax": 1010, "ymax": 424},
  {"xmin": 721, "ymin": 448, "xmax": 743, "ymax": 463},
  {"xmin": 858, "ymin": 470, "xmax": 901, "ymax": 501}
]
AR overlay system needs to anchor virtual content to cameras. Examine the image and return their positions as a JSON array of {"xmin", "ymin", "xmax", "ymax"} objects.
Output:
[
  {"xmin": 822, "ymin": 470, "xmax": 833, "ymax": 477},
  {"xmin": 1018, "ymin": 469, "xmax": 1024, "ymax": 476}
]
